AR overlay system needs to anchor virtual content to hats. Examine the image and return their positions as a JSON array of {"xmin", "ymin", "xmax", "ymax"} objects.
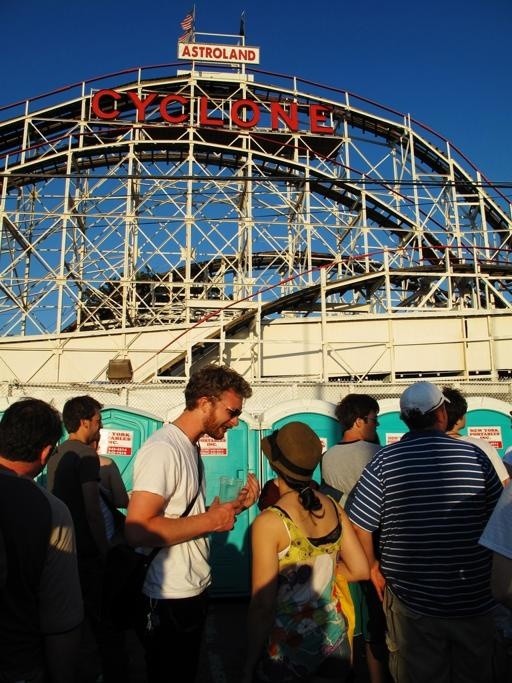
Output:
[
  {"xmin": 258, "ymin": 420, "xmax": 322, "ymax": 481},
  {"xmin": 400, "ymin": 381, "xmax": 451, "ymax": 415}
]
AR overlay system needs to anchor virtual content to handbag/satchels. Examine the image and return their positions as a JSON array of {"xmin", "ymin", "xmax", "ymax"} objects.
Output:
[{"xmin": 94, "ymin": 545, "xmax": 150, "ymax": 632}]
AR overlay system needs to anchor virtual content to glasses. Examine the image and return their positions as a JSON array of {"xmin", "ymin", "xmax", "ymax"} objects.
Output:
[
  {"xmin": 209, "ymin": 394, "xmax": 242, "ymax": 418},
  {"xmin": 364, "ymin": 417, "xmax": 378, "ymax": 423}
]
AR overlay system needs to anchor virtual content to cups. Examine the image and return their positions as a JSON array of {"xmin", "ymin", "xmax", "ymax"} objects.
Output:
[{"xmin": 219, "ymin": 475, "xmax": 243, "ymax": 505}]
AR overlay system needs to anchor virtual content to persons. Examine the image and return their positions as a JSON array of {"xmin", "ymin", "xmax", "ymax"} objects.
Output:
[{"xmin": 2, "ymin": 360, "xmax": 508, "ymax": 680}]
